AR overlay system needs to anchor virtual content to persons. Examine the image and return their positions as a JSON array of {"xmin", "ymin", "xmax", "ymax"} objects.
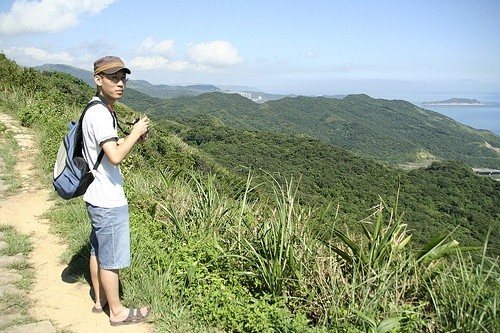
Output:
[{"xmin": 82, "ymin": 56, "xmax": 151, "ymax": 326}]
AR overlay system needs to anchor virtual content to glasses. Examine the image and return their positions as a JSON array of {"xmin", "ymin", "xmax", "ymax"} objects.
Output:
[{"xmin": 100, "ymin": 72, "xmax": 129, "ymax": 86}]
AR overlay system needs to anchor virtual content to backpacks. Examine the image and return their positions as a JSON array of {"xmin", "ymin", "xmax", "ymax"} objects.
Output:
[{"xmin": 52, "ymin": 100, "xmax": 118, "ymax": 200}]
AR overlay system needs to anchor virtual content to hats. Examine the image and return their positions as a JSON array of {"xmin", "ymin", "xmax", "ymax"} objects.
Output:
[{"xmin": 94, "ymin": 56, "xmax": 131, "ymax": 76}]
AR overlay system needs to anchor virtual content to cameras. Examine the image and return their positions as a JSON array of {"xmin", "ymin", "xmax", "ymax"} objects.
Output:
[{"xmin": 134, "ymin": 117, "xmax": 149, "ymax": 139}]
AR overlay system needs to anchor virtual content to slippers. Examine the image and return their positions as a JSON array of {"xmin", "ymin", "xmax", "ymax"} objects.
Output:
[
  {"xmin": 92, "ymin": 301, "xmax": 109, "ymax": 314},
  {"xmin": 110, "ymin": 305, "xmax": 150, "ymax": 325}
]
